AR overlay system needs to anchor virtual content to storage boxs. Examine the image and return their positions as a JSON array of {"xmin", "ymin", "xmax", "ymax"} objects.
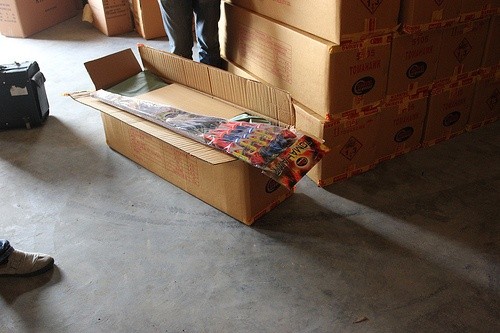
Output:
[
  {"xmin": 130, "ymin": 0, "xmax": 195, "ymax": 40},
  {"xmin": 66, "ymin": 43, "xmax": 297, "ymax": 226},
  {"xmin": 216, "ymin": 0, "xmax": 500, "ymax": 188},
  {"xmin": 0, "ymin": 0, "xmax": 87, "ymax": 38},
  {"xmin": 88, "ymin": 0, "xmax": 134, "ymax": 37}
]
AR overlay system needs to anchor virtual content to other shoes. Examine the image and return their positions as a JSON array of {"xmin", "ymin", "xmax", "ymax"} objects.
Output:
[{"xmin": 0, "ymin": 240, "xmax": 54, "ymax": 276}]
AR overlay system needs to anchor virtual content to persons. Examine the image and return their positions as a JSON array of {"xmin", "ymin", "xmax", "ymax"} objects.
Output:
[
  {"xmin": 0, "ymin": 239, "xmax": 54, "ymax": 277},
  {"xmin": 157, "ymin": 0, "xmax": 221, "ymax": 68}
]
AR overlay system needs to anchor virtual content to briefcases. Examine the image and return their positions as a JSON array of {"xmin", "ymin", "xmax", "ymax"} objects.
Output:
[{"xmin": 0, "ymin": 60, "xmax": 50, "ymax": 129}]
